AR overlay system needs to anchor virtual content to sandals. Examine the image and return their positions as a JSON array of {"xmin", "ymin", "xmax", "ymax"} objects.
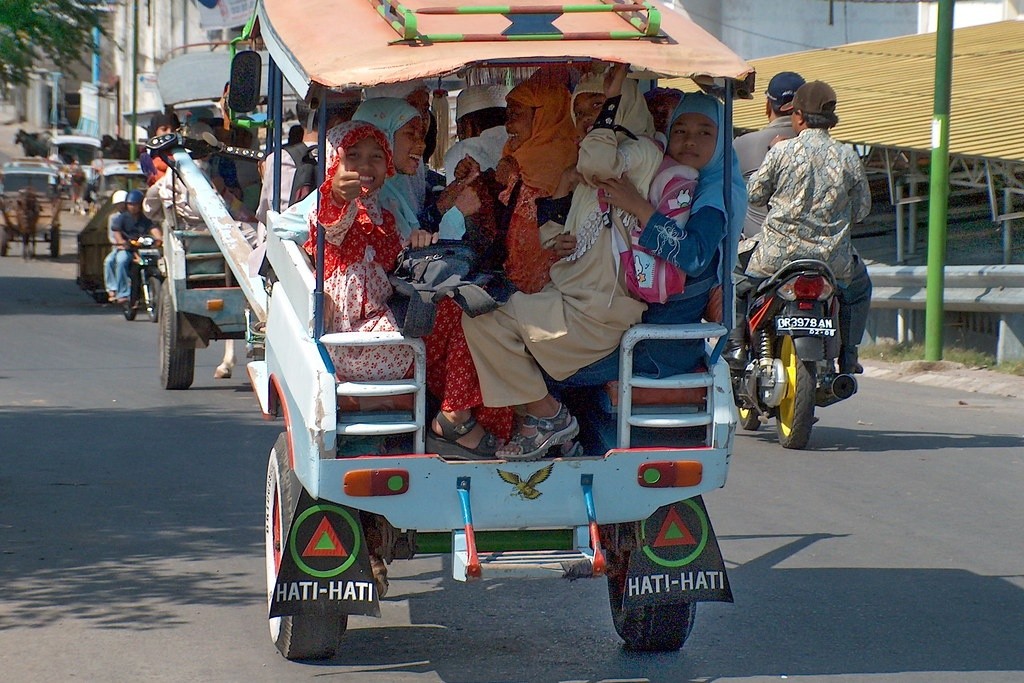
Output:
[
  {"xmin": 494, "ymin": 398, "xmax": 579, "ymax": 461},
  {"xmin": 426, "ymin": 410, "xmax": 508, "ymax": 460}
]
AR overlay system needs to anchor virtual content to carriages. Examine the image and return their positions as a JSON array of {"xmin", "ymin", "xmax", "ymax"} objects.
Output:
[
  {"xmin": 0, "ymin": 162, "xmax": 61, "ymax": 261},
  {"xmin": 48, "ymin": 135, "xmax": 149, "ymax": 217},
  {"xmin": 159, "ymin": 0, "xmax": 759, "ymax": 665}
]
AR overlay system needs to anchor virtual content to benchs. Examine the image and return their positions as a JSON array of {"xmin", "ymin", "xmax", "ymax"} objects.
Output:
[
  {"xmin": 265, "ymin": 208, "xmax": 427, "ymax": 457},
  {"xmin": 164, "ymin": 199, "xmax": 233, "ymax": 290},
  {"xmin": 595, "ymin": 323, "xmax": 728, "ymax": 452}
]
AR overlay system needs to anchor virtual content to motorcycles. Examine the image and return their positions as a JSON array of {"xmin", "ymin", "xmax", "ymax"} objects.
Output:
[
  {"xmin": 734, "ymin": 258, "xmax": 858, "ymax": 449},
  {"xmin": 108, "ymin": 224, "xmax": 162, "ymax": 324}
]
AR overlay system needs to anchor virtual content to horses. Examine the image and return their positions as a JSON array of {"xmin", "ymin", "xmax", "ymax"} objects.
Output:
[
  {"xmin": 16, "ymin": 188, "xmax": 39, "ymax": 261},
  {"xmin": 14, "ymin": 128, "xmax": 52, "ymax": 158}
]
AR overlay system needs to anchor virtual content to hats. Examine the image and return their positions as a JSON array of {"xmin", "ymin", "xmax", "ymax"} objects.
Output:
[
  {"xmin": 765, "ymin": 72, "xmax": 806, "ymax": 102},
  {"xmin": 151, "ymin": 111, "xmax": 181, "ymax": 137},
  {"xmin": 455, "ymin": 84, "xmax": 508, "ymax": 119},
  {"xmin": 780, "ymin": 80, "xmax": 837, "ymax": 115}
]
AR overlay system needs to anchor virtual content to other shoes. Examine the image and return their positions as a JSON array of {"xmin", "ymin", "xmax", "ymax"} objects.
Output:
[
  {"xmin": 840, "ymin": 346, "xmax": 858, "ymax": 373},
  {"xmin": 837, "ymin": 358, "xmax": 863, "ymax": 374},
  {"xmin": 722, "ymin": 347, "xmax": 749, "ymax": 370}
]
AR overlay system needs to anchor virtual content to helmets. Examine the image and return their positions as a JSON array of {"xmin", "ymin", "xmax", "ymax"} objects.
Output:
[
  {"xmin": 112, "ymin": 189, "xmax": 128, "ymax": 205},
  {"xmin": 125, "ymin": 190, "xmax": 144, "ymax": 204}
]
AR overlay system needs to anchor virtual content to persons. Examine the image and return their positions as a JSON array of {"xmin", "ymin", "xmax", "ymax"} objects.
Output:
[
  {"xmin": 66, "ymin": 153, "xmax": 88, "ymax": 215},
  {"xmin": 719, "ymin": 79, "xmax": 872, "ymax": 378},
  {"xmin": 111, "ymin": 190, "xmax": 163, "ymax": 304},
  {"xmin": 256, "ymin": 60, "xmax": 750, "ymax": 460},
  {"xmin": 536, "ymin": 89, "xmax": 748, "ymax": 389},
  {"xmin": 141, "ymin": 105, "xmax": 261, "ymax": 249},
  {"xmin": 733, "ymin": 71, "xmax": 807, "ymax": 240},
  {"xmin": 102, "ymin": 190, "xmax": 128, "ymax": 301}
]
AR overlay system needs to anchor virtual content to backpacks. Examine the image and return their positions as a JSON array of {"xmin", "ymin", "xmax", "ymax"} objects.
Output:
[
  {"xmin": 598, "ymin": 154, "xmax": 702, "ymax": 304},
  {"xmin": 388, "ymin": 240, "xmax": 512, "ymax": 337}
]
